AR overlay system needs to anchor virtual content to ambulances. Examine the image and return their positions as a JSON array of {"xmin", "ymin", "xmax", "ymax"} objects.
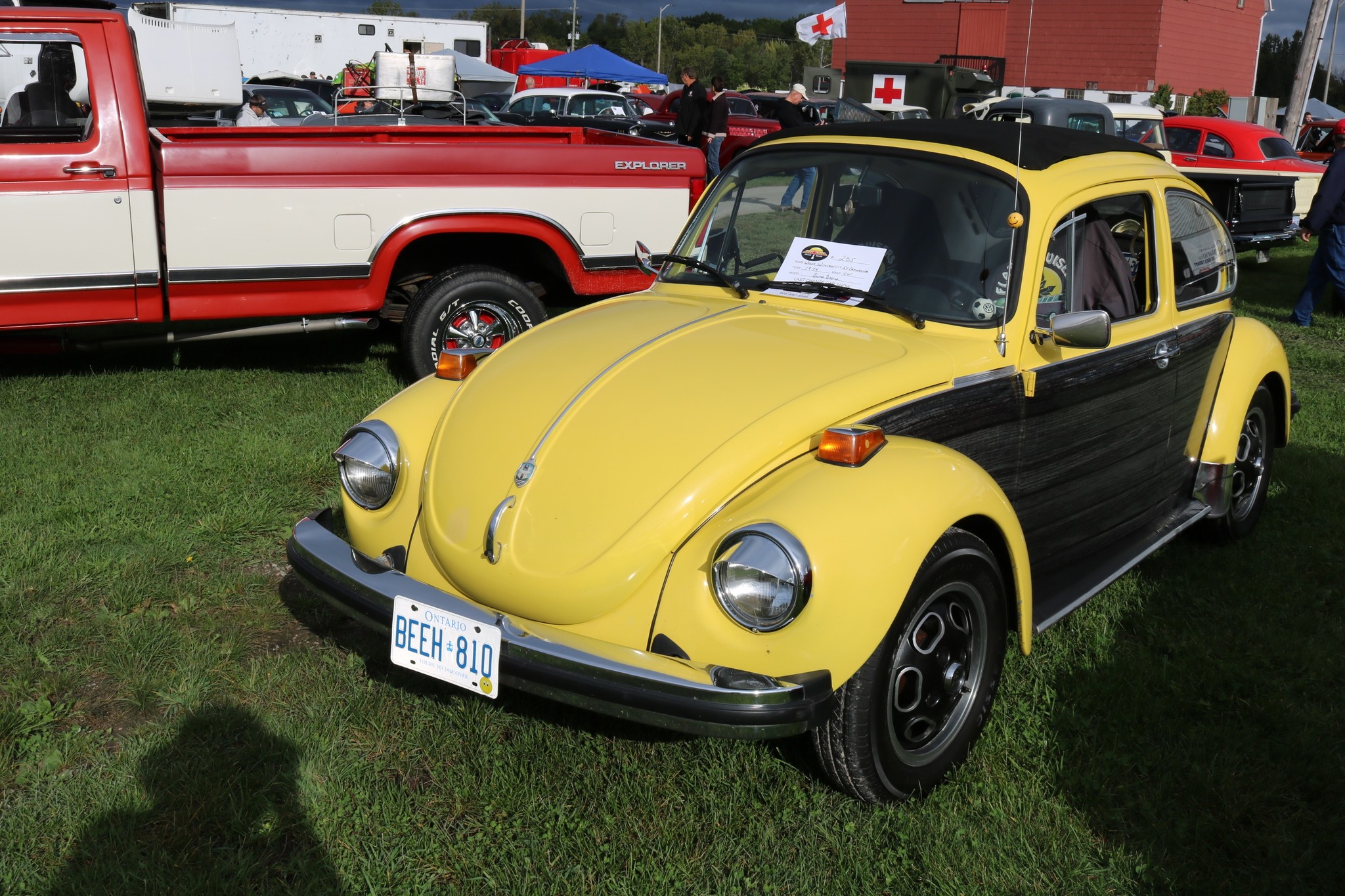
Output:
[{"xmin": 802, "ymin": 59, "xmax": 1001, "ymax": 121}]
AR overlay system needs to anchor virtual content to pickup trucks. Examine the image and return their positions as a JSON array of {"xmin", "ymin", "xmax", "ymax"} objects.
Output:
[{"xmin": 0, "ymin": 6, "xmax": 709, "ymax": 382}]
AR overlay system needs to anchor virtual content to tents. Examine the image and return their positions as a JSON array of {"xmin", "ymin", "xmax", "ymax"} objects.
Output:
[
  {"xmin": 428, "ymin": 48, "xmax": 518, "ymax": 98},
  {"xmin": 511, "ymin": 44, "xmax": 669, "ymax": 118}
]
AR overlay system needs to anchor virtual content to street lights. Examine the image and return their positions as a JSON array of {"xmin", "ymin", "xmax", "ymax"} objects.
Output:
[{"xmin": 655, "ymin": 3, "xmax": 678, "ymax": 92}]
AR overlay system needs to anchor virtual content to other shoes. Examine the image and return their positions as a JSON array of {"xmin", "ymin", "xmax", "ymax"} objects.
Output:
[
  {"xmin": 780, "ymin": 204, "xmax": 797, "ymax": 211},
  {"xmin": 799, "ymin": 209, "xmax": 806, "ymax": 214}
]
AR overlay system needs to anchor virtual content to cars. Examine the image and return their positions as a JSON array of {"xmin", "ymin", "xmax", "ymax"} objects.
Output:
[
  {"xmin": 958, "ymin": 98, "xmax": 1173, "ymax": 166},
  {"xmin": 284, "ymin": 119, "xmax": 1306, "ymax": 813},
  {"xmin": 1136, "ymin": 115, "xmax": 1329, "ymax": 173},
  {"xmin": 148, "ymin": 87, "xmax": 897, "ymax": 181},
  {"xmin": 1275, "ymin": 114, "xmax": 1340, "ymax": 161}
]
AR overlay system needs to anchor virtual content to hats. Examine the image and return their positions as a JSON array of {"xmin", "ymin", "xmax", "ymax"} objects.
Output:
[
  {"xmin": 327, "ymin": 76, "xmax": 332, "ymax": 80},
  {"xmin": 249, "ymin": 100, "xmax": 270, "ymax": 110},
  {"xmin": 792, "ymin": 83, "xmax": 809, "ymax": 100},
  {"xmin": 1331, "ymin": 119, "xmax": 1345, "ymax": 134},
  {"xmin": 310, "ymin": 72, "xmax": 315, "ymax": 75}
]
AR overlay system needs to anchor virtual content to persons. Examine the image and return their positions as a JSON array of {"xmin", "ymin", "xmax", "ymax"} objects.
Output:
[
  {"xmin": 236, "ymin": 94, "xmax": 280, "ymax": 126},
  {"xmin": 707, "ymin": 77, "xmax": 728, "ymax": 185},
  {"xmin": 1289, "ymin": 119, "xmax": 1345, "ymax": 328},
  {"xmin": 672, "ymin": 67, "xmax": 707, "ymax": 149},
  {"xmin": 310, "ymin": 72, "xmax": 317, "ymax": 79},
  {"xmin": 319, "ymin": 74, "xmax": 333, "ymax": 80},
  {"xmin": 776, "ymin": 83, "xmax": 824, "ymax": 213},
  {"xmin": 1296, "ymin": 112, "xmax": 1320, "ymax": 152}
]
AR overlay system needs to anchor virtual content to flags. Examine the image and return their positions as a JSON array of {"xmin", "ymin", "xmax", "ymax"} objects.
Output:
[{"xmin": 796, "ymin": 3, "xmax": 847, "ymax": 46}]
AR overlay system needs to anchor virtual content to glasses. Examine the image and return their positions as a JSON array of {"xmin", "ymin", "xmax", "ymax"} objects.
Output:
[
  {"xmin": 710, "ymin": 83, "xmax": 712, "ymax": 86},
  {"xmin": 680, "ymin": 74, "xmax": 685, "ymax": 76}
]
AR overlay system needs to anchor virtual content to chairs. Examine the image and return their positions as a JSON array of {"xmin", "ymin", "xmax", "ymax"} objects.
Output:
[
  {"xmin": 830, "ymin": 187, "xmax": 1215, "ymax": 337},
  {"xmin": 1, "ymin": 41, "xmax": 85, "ymax": 126}
]
